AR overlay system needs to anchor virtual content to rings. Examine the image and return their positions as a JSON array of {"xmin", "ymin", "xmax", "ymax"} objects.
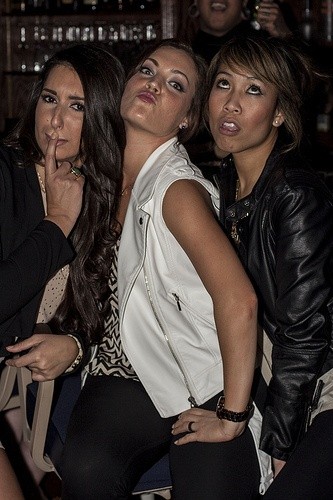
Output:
[
  {"xmin": 189, "ymin": 422, "xmax": 193, "ymax": 433},
  {"xmin": 70, "ymin": 168, "xmax": 81, "ymax": 177}
]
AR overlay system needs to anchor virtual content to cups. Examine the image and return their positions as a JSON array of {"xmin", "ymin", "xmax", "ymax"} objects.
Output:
[{"xmin": 16, "ymin": 0, "xmax": 161, "ymax": 72}]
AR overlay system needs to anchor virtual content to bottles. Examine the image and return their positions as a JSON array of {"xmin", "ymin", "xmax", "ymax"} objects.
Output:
[{"xmin": 300, "ymin": 0, "xmax": 333, "ymax": 43}]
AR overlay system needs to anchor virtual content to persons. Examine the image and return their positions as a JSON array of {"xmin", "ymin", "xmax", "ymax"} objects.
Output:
[
  {"xmin": 182, "ymin": 0, "xmax": 296, "ymax": 65},
  {"xmin": 46, "ymin": 39, "xmax": 261, "ymax": 500},
  {"xmin": 0, "ymin": 45, "xmax": 127, "ymax": 500},
  {"xmin": 199, "ymin": 35, "xmax": 333, "ymax": 500}
]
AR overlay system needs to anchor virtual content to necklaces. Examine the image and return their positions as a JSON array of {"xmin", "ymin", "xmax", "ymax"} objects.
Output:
[
  {"xmin": 37, "ymin": 171, "xmax": 46, "ymax": 193},
  {"xmin": 236, "ymin": 180, "xmax": 240, "ymax": 200}
]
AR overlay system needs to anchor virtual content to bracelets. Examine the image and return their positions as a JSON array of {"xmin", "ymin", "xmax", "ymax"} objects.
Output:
[
  {"xmin": 65, "ymin": 334, "xmax": 83, "ymax": 373},
  {"xmin": 217, "ymin": 396, "xmax": 255, "ymax": 422}
]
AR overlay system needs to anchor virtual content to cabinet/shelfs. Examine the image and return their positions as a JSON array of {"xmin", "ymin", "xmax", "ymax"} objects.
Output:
[{"xmin": 0, "ymin": 0, "xmax": 178, "ymax": 127}]
